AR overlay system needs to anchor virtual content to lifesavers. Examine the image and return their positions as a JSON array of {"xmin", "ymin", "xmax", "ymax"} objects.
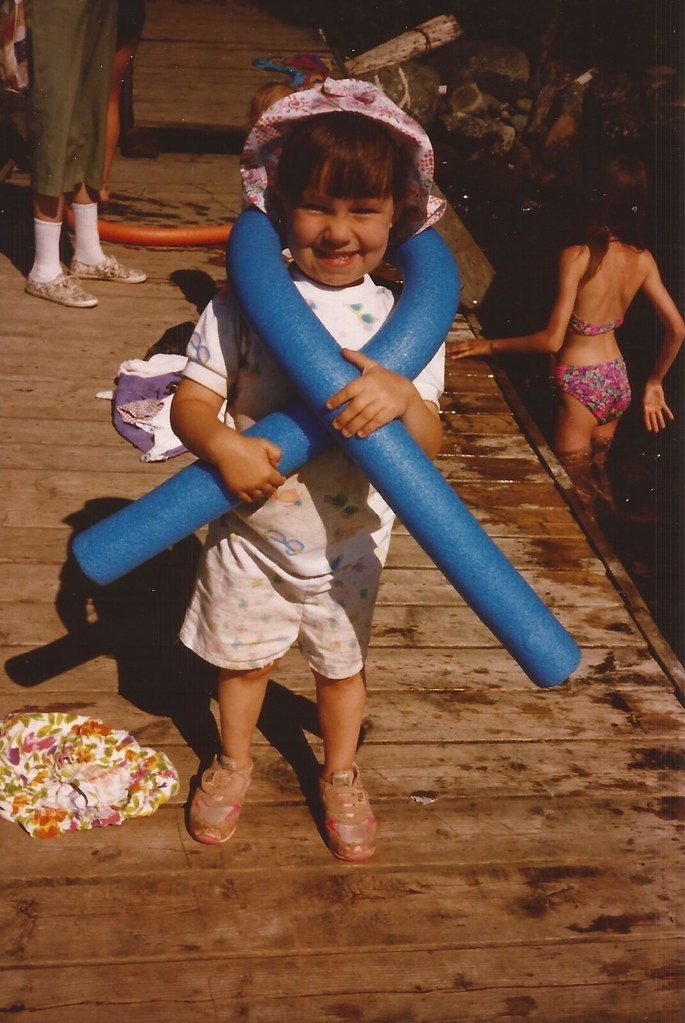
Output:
[{"xmin": 67, "ymin": 192, "xmax": 587, "ymax": 688}]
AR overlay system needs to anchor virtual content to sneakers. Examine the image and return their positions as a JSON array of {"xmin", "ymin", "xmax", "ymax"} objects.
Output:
[
  {"xmin": 24, "ymin": 271, "xmax": 100, "ymax": 307},
  {"xmin": 68, "ymin": 252, "xmax": 146, "ymax": 284},
  {"xmin": 318, "ymin": 765, "xmax": 375, "ymax": 861},
  {"xmin": 188, "ymin": 750, "xmax": 254, "ymax": 846}
]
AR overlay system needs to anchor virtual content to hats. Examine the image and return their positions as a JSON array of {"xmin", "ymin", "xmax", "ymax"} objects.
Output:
[{"xmin": 240, "ymin": 79, "xmax": 447, "ymax": 237}]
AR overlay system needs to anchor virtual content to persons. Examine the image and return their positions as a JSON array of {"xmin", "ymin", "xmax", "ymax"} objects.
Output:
[
  {"xmin": 170, "ymin": 77, "xmax": 445, "ymax": 861},
  {"xmin": 23, "ymin": 1, "xmax": 148, "ymax": 306},
  {"xmin": 447, "ymin": 155, "xmax": 685, "ymax": 452},
  {"xmin": 248, "ymin": 82, "xmax": 294, "ymax": 125}
]
